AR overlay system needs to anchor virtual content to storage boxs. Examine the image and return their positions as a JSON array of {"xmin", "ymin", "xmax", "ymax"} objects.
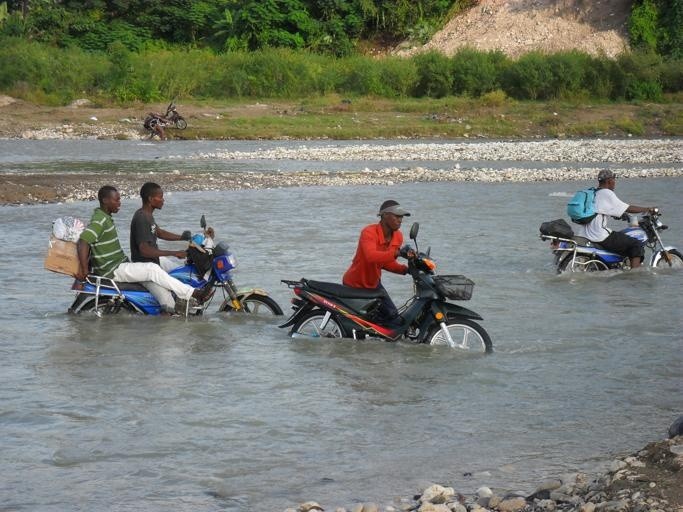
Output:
[{"xmin": 44, "ymin": 237, "xmax": 80, "ymax": 277}]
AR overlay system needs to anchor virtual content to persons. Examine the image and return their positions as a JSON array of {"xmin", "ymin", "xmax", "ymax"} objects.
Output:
[
  {"xmin": 129, "ymin": 183, "xmax": 190, "ymax": 315},
  {"xmin": 343, "ymin": 200, "xmax": 419, "ymax": 320},
  {"xmin": 72, "ymin": 186, "xmax": 215, "ymax": 318},
  {"xmin": 579, "ymin": 168, "xmax": 657, "ymax": 268},
  {"xmin": 148, "ymin": 122, "xmax": 165, "ymax": 141}
]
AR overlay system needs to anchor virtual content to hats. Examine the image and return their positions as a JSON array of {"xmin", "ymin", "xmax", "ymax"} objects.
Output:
[
  {"xmin": 598, "ymin": 170, "xmax": 616, "ymax": 182},
  {"xmin": 376, "ymin": 204, "xmax": 411, "ymax": 218}
]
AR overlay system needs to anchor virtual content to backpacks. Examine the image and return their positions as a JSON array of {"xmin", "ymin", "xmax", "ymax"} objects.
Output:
[{"xmin": 567, "ymin": 186, "xmax": 608, "ymax": 224}]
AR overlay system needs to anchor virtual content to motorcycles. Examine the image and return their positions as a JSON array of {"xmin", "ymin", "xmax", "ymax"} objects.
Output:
[
  {"xmin": 143, "ymin": 101, "xmax": 189, "ymax": 131},
  {"xmin": 277, "ymin": 221, "xmax": 493, "ymax": 351},
  {"xmin": 63, "ymin": 211, "xmax": 284, "ymax": 315},
  {"xmin": 535, "ymin": 206, "xmax": 683, "ymax": 276}
]
